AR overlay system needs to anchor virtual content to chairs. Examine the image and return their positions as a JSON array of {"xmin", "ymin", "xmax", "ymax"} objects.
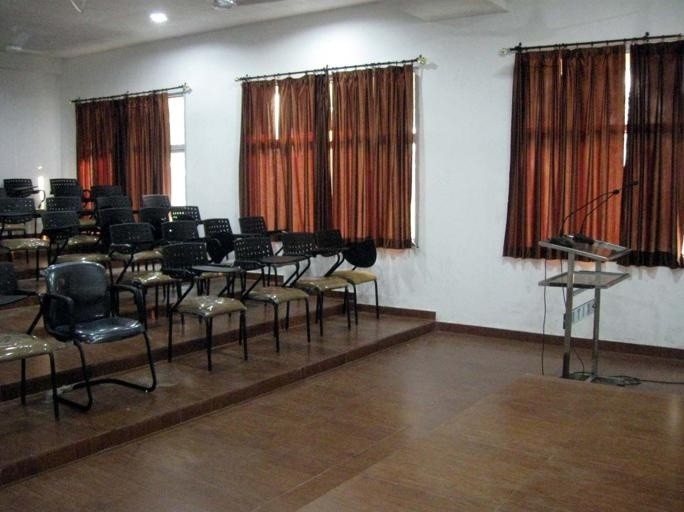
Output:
[
  {"xmin": 1, "ymin": 332, "xmax": 61, "ymax": 421},
  {"xmin": 38, "ymin": 262, "xmax": 156, "ymax": 413},
  {"xmin": 1, "ymin": 178, "xmax": 379, "ymax": 337},
  {"xmin": 159, "ymin": 242, "xmax": 248, "ymax": 371},
  {"xmin": 230, "ymin": 237, "xmax": 312, "ymax": 353}
]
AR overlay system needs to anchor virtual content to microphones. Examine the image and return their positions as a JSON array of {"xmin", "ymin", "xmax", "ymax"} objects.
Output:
[
  {"xmin": 551, "ymin": 189, "xmax": 619, "ymax": 247},
  {"xmin": 573, "ymin": 180, "xmax": 638, "ymax": 244}
]
